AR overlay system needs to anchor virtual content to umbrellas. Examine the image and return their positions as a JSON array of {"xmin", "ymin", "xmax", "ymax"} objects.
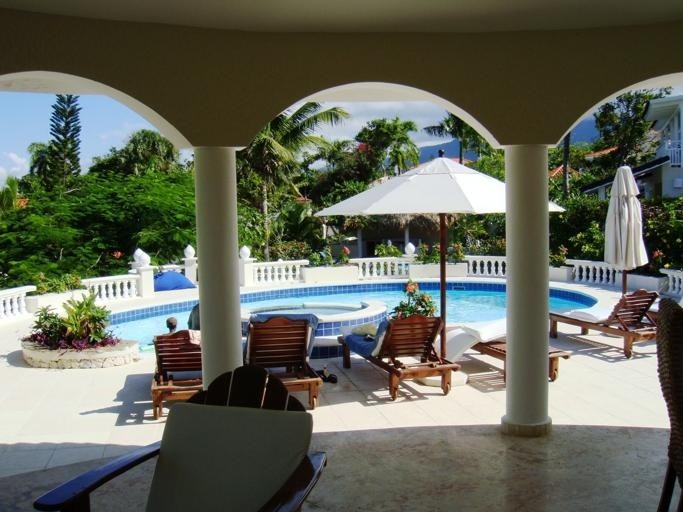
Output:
[
  {"xmin": 604, "ymin": 166, "xmax": 649, "ymax": 295},
  {"xmin": 314, "ymin": 149, "xmax": 566, "ymax": 360}
]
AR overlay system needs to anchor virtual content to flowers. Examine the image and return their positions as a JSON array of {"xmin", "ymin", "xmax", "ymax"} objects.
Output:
[
  {"xmin": 407, "ymin": 242, "xmax": 465, "ymax": 265},
  {"xmin": 310, "ymin": 245, "xmax": 350, "ymax": 268},
  {"xmin": 383, "ymin": 277, "xmax": 441, "ymax": 323}
]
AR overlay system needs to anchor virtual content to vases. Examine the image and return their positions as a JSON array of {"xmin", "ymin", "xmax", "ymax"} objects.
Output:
[
  {"xmin": 407, "ymin": 262, "xmax": 468, "ymax": 282},
  {"xmin": 299, "ymin": 264, "xmax": 359, "ymax": 285}
]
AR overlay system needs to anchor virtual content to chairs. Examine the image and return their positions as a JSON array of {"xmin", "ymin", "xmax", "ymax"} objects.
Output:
[
  {"xmin": 460, "ymin": 326, "xmax": 571, "ymax": 392},
  {"xmin": 338, "ymin": 312, "xmax": 461, "ymax": 401},
  {"xmin": 549, "ymin": 290, "xmax": 659, "ymax": 359},
  {"xmin": 150, "ymin": 330, "xmax": 203, "ymax": 419},
  {"xmin": 30, "ymin": 368, "xmax": 327, "ymax": 512},
  {"xmin": 241, "ymin": 313, "xmax": 321, "ymax": 405},
  {"xmin": 642, "ymin": 293, "xmax": 683, "ymax": 321}
]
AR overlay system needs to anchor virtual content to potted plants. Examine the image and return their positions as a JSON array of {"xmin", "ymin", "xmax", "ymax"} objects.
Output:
[{"xmin": 23, "ymin": 271, "xmax": 90, "ymax": 314}]
